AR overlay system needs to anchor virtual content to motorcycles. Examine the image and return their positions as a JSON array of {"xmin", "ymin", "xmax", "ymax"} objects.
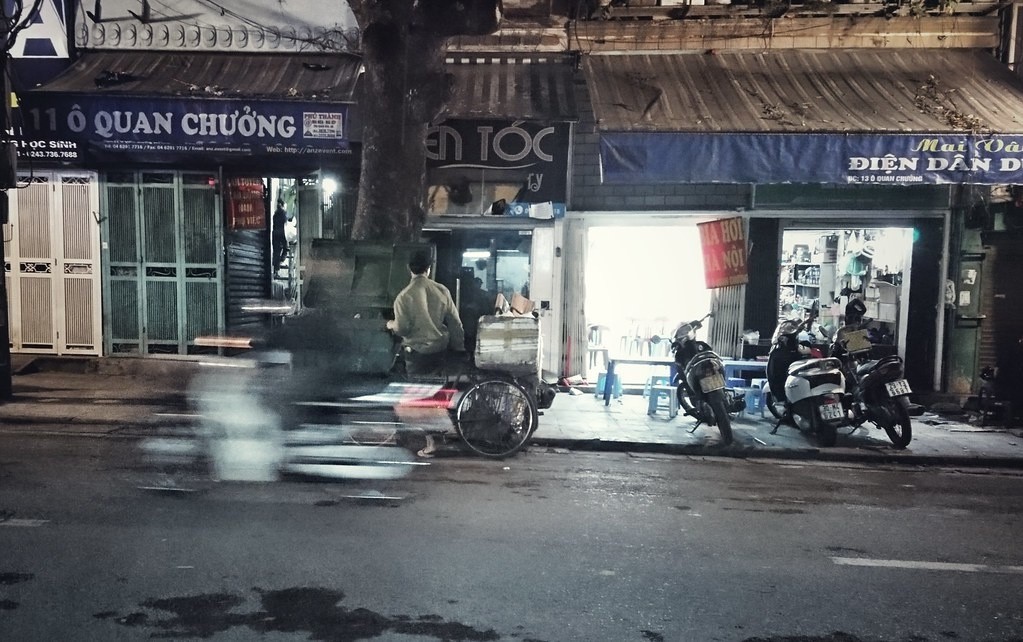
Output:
[
  {"xmin": 766, "ymin": 300, "xmax": 847, "ymax": 447},
  {"xmin": 820, "ymin": 298, "xmax": 913, "ymax": 448},
  {"xmin": 650, "ymin": 311, "xmax": 748, "ymax": 444}
]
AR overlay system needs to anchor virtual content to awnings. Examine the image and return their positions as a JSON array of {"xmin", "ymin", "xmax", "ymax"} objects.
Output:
[
  {"xmin": 583, "ymin": 53, "xmax": 1023, "ymax": 186},
  {"xmin": 0, "ymin": 49, "xmax": 580, "ymax": 184}
]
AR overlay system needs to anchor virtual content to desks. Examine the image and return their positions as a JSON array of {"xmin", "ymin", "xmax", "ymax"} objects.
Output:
[
  {"xmin": 602, "ymin": 357, "xmax": 679, "ymax": 406},
  {"xmin": 723, "ymin": 360, "xmax": 767, "ymax": 381}
]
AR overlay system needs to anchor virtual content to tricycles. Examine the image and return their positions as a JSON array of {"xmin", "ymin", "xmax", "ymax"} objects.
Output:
[{"xmin": 338, "ymin": 325, "xmax": 538, "ymax": 460}]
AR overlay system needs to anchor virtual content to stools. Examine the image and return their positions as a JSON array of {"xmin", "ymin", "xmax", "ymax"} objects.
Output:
[
  {"xmin": 643, "ymin": 377, "xmax": 651, "ymax": 398},
  {"xmin": 594, "ymin": 372, "xmax": 619, "ymax": 399},
  {"xmin": 648, "ymin": 375, "xmax": 678, "ymax": 417},
  {"xmin": 727, "ymin": 377, "xmax": 768, "ymax": 418}
]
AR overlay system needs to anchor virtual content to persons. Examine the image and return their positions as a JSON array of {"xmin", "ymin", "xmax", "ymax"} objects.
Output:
[
  {"xmin": 385, "ymin": 251, "xmax": 465, "ymax": 457},
  {"xmin": 273, "ymin": 199, "xmax": 288, "ymax": 278}
]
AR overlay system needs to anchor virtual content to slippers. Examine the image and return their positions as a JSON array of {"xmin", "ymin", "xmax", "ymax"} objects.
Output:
[{"xmin": 417, "ymin": 446, "xmax": 436, "ymax": 457}]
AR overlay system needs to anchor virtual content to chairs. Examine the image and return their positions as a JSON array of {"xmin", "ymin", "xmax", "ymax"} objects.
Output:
[{"xmin": 587, "ymin": 318, "xmax": 669, "ymax": 371}]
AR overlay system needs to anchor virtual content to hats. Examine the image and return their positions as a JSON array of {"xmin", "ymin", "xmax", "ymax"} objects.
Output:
[{"xmin": 407, "ymin": 251, "xmax": 435, "ymax": 266}]
